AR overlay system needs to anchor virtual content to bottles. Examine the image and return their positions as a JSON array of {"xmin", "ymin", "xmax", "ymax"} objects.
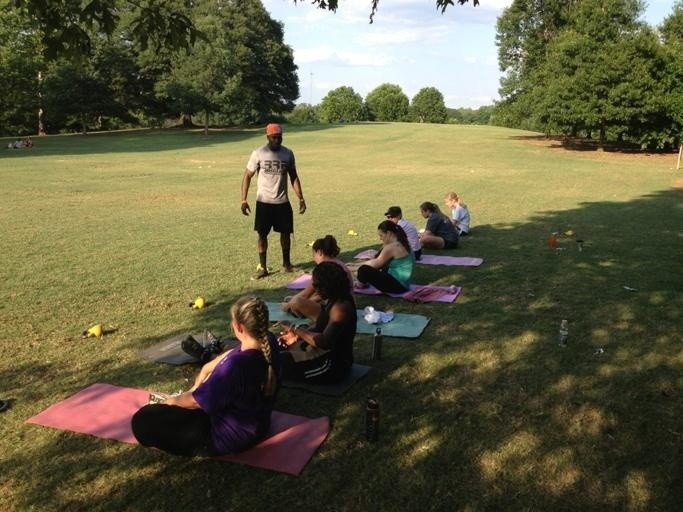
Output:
[
  {"xmin": 371, "ymin": 327, "xmax": 384, "ymax": 363},
  {"xmin": 365, "ymin": 397, "xmax": 381, "ymax": 445},
  {"xmin": 557, "ymin": 320, "xmax": 570, "ymax": 349},
  {"xmin": 546, "ymin": 230, "xmax": 587, "ymax": 259}
]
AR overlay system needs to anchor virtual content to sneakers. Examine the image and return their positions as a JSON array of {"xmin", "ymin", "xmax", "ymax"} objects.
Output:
[
  {"xmin": 251, "ymin": 267, "xmax": 269, "ymax": 280},
  {"xmin": 279, "ymin": 264, "xmax": 301, "ymax": 274},
  {"xmin": 202, "ymin": 327, "xmax": 222, "ymax": 352},
  {"xmin": 148, "ymin": 390, "xmax": 185, "ymax": 405},
  {"xmin": 179, "ymin": 335, "xmax": 210, "ymax": 362}
]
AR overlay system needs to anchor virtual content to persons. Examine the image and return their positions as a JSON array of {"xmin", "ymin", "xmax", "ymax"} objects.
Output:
[
  {"xmin": 417, "ymin": 201, "xmax": 458, "ymax": 250},
  {"xmin": 355, "ymin": 220, "xmax": 415, "ymax": 291},
  {"xmin": 443, "ymin": 192, "xmax": 470, "ymax": 237},
  {"xmin": 280, "ymin": 234, "xmax": 353, "ymax": 323},
  {"xmin": 130, "ymin": 293, "xmax": 278, "ymax": 457},
  {"xmin": 367, "ymin": 204, "xmax": 421, "ymax": 261},
  {"xmin": 181, "ymin": 260, "xmax": 355, "ymax": 383},
  {"xmin": 239, "ymin": 124, "xmax": 306, "ymax": 280}
]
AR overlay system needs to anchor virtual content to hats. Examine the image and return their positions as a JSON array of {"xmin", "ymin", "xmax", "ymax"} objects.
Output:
[
  {"xmin": 265, "ymin": 123, "xmax": 282, "ymax": 137},
  {"xmin": 383, "ymin": 206, "xmax": 401, "ymax": 218}
]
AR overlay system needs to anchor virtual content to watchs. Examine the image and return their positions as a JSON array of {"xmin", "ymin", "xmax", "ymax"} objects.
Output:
[{"xmin": 293, "ymin": 324, "xmax": 299, "ymax": 330}]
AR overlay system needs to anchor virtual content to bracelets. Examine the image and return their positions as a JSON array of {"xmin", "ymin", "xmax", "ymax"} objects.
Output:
[
  {"xmin": 288, "ymin": 323, "xmax": 294, "ymax": 330},
  {"xmin": 241, "ymin": 200, "xmax": 246, "ymax": 203},
  {"xmin": 299, "ymin": 199, "xmax": 304, "ymax": 202}
]
